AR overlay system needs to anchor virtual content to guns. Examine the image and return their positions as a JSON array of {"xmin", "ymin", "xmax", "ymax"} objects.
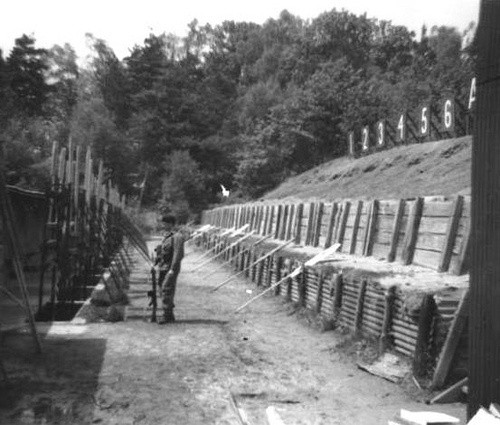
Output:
[{"xmin": 147, "ymin": 253, "xmax": 156, "ymax": 320}]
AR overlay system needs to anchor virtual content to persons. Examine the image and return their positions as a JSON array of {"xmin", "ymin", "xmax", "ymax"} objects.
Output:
[{"xmin": 158, "ymin": 214, "xmax": 185, "ymax": 320}]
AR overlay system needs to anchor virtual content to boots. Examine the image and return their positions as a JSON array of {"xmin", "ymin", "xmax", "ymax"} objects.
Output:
[{"xmin": 159, "ymin": 307, "xmax": 174, "ymax": 323}]
